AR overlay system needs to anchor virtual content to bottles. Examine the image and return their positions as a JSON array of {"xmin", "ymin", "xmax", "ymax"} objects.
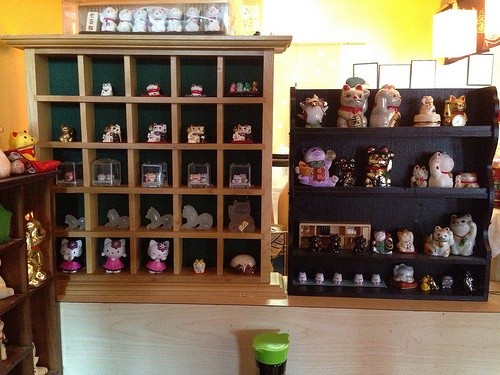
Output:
[{"xmin": 251, "ymin": 331, "xmax": 290, "ymax": 375}]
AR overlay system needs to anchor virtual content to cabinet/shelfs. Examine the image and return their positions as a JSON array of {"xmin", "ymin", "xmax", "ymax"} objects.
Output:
[
  {"xmin": 298, "ymin": 221, "xmax": 371, "ymax": 250},
  {"xmin": 0, "ymin": 170, "xmax": 63, "ymax": 375},
  {"xmin": 287, "ymin": 87, "xmax": 500, "ymax": 302},
  {"xmin": 0, "ymin": 34, "xmax": 292, "ymax": 306}
]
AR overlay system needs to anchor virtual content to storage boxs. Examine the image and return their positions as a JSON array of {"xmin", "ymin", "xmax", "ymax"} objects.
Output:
[
  {"xmin": 228, "ymin": 163, "xmax": 251, "ymax": 189},
  {"xmin": 56, "ymin": 161, "xmax": 83, "ymax": 186},
  {"xmin": 142, "ymin": 162, "xmax": 168, "ymax": 187},
  {"xmin": 188, "ymin": 162, "xmax": 210, "ymax": 188},
  {"xmin": 91, "ymin": 159, "xmax": 121, "ymax": 187}
]
